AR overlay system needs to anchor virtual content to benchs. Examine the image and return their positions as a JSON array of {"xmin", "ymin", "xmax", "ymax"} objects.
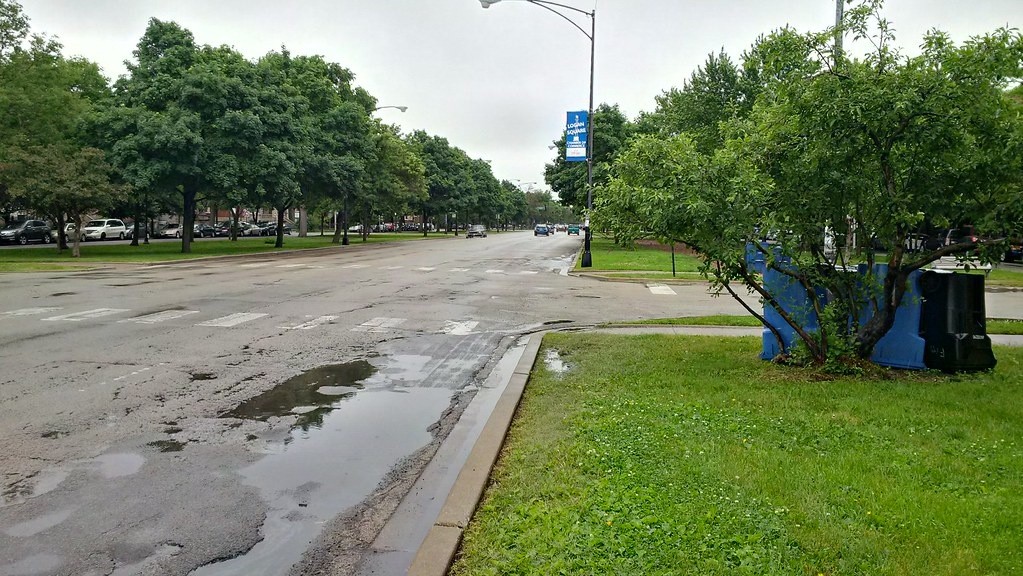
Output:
[{"xmin": 932, "ymin": 256, "xmax": 992, "ymax": 278}]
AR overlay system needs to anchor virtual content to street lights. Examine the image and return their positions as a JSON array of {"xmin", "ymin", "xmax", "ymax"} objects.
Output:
[
  {"xmin": 480, "ymin": 0, "xmax": 595, "ymax": 268},
  {"xmin": 341, "ymin": 105, "xmax": 408, "ymax": 245}
]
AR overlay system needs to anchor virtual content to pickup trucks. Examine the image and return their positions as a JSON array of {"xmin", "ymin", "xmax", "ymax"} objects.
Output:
[{"xmin": 567, "ymin": 224, "xmax": 579, "ymax": 235}]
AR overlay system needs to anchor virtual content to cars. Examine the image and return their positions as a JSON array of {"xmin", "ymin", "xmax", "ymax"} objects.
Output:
[
  {"xmin": 451, "ymin": 222, "xmax": 470, "ymax": 229},
  {"xmin": 124, "ymin": 220, "xmax": 292, "ymax": 239},
  {"xmin": 465, "ymin": 225, "xmax": 487, "ymax": 238},
  {"xmin": 903, "ymin": 233, "xmax": 930, "ymax": 253},
  {"xmin": 50, "ymin": 223, "xmax": 87, "ymax": 242},
  {"xmin": 556, "ymin": 225, "xmax": 567, "ymax": 232},
  {"xmin": 547, "ymin": 224, "xmax": 555, "ymax": 234},
  {"xmin": 348, "ymin": 222, "xmax": 434, "ymax": 233},
  {"xmin": 958, "ymin": 225, "xmax": 1023, "ymax": 263},
  {"xmin": 919, "ymin": 229, "xmax": 961, "ymax": 253},
  {"xmin": 84, "ymin": 219, "xmax": 127, "ymax": 240},
  {"xmin": 754, "ymin": 227, "xmax": 803, "ymax": 241},
  {"xmin": 0, "ymin": 220, "xmax": 52, "ymax": 245},
  {"xmin": 873, "ymin": 236, "xmax": 901, "ymax": 251},
  {"xmin": 534, "ymin": 224, "xmax": 549, "ymax": 236}
]
